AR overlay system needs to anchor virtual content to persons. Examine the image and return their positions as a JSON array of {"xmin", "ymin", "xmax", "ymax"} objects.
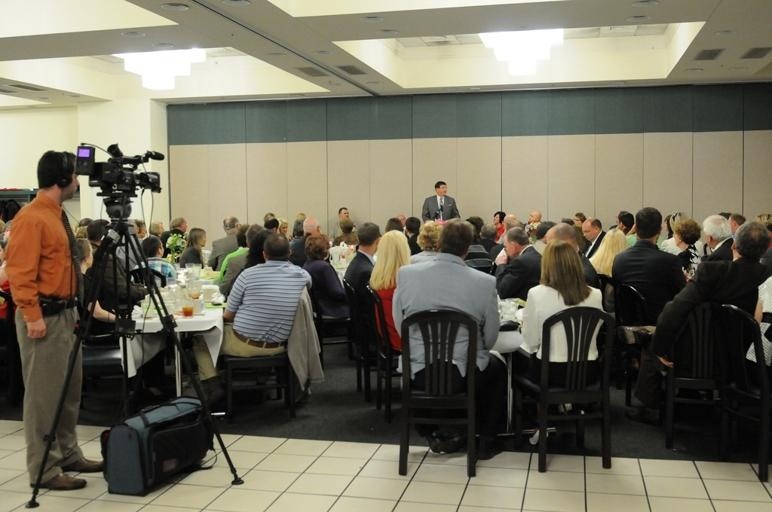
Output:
[
  {"xmin": 0, "ymin": 200, "xmax": 772, "ymax": 448},
  {"xmin": 4, "ymin": 150, "xmax": 103, "ymax": 490},
  {"xmin": 422, "ymin": 181, "xmax": 459, "ymax": 221}
]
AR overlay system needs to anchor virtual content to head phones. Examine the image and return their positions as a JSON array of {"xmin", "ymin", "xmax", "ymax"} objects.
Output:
[{"xmin": 55, "ymin": 152, "xmax": 70, "ymax": 187}]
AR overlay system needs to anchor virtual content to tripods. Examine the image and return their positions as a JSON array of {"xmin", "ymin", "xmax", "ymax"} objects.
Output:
[{"xmin": 25, "ymin": 198, "xmax": 244, "ymax": 508}]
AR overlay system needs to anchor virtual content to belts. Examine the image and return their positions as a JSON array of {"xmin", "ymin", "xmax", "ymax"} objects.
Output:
[
  {"xmin": 232, "ymin": 326, "xmax": 288, "ymax": 350},
  {"xmin": 38, "ymin": 290, "xmax": 79, "ymax": 310}
]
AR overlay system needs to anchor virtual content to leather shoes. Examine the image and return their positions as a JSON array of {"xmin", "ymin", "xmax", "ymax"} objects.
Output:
[
  {"xmin": 59, "ymin": 455, "xmax": 106, "ymax": 476},
  {"xmin": 28, "ymin": 473, "xmax": 88, "ymax": 493},
  {"xmin": 427, "ymin": 429, "xmax": 467, "ymax": 457}
]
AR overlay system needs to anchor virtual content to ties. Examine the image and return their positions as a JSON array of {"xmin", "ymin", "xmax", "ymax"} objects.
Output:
[
  {"xmin": 439, "ymin": 197, "xmax": 445, "ymax": 212},
  {"xmin": 60, "ymin": 207, "xmax": 86, "ymax": 310}
]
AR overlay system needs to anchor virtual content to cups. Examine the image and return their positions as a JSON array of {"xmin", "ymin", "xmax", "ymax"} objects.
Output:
[
  {"xmin": 328, "ymin": 242, "xmax": 360, "ymax": 266},
  {"xmin": 498, "ymin": 296, "xmax": 518, "ymax": 323},
  {"xmin": 136, "ymin": 256, "xmax": 209, "ymax": 319}
]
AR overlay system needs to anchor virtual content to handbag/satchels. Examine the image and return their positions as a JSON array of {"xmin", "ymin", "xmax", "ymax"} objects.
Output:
[{"xmin": 98, "ymin": 393, "xmax": 215, "ymax": 498}]
